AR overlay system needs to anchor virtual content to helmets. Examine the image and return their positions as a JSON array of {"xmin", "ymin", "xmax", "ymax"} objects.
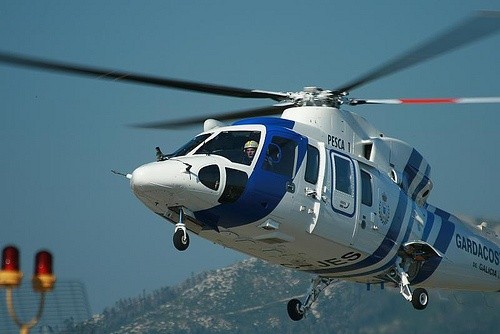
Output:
[{"xmin": 243, "ymin": 140, "xmax": 259, "ymax": 148}]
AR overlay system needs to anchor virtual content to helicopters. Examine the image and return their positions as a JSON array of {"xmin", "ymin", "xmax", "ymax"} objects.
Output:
[{"xmin": 0, "ymin": 12, "xmax": 500, "ymax": 322}]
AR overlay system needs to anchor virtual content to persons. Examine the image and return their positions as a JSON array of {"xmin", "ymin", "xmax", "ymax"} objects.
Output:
[{"xmin": 242, "ymin": 140, "xmax": 269, "ymax": 170}]
